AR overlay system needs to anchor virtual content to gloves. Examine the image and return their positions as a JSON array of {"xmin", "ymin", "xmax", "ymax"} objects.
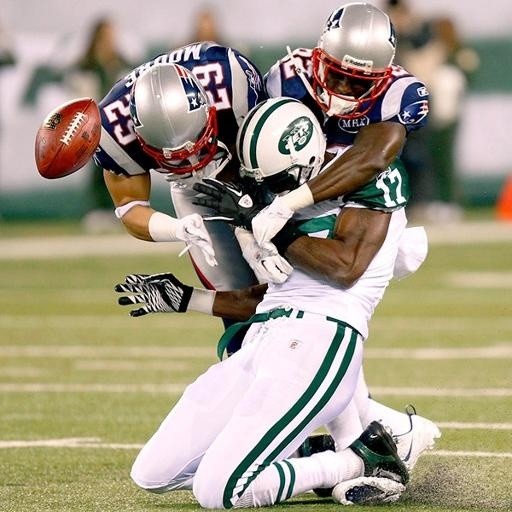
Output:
[
  {"xmin": 111, "ymin": 270, "xmax": 194, "ymax": 319},
  {"xmin": 231, "ymin": 224, "xmax": 294, "ymax": 287},
  {"xmin": 173, "ymin": 212, "xmax": 218, "ymax": 269},
  {"xmin": 250, "ymin": 181, "xmax": 317, "ymax": 257},
  {"xmin": 189, "ymin": 174, "xmax": 270, "ymax": 235}
]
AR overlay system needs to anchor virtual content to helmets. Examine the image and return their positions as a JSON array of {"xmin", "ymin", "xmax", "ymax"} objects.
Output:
[
  {"xmin": 128, "ymin": 62, "xmax": 214, "ymax": 157},
  {"xmin": 313, "ymin": 0, "xmax": 398, "ymax": 74},
  {"xmin": 234, "ymin": 96, "xmax": 328, "ymax": 186}
]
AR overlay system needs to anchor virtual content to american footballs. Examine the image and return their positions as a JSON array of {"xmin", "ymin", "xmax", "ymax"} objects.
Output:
[{"xmin": 36, "ymin": 98, "xmax": 102, "ymax": 178}]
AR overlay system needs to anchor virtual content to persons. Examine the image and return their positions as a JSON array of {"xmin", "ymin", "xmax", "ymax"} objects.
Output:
[
  {"xmin": 2, "ymin": 1, "xmax": 470, "ymax": 236},
  {"xmin": 88, "ymin": 3, "xmax": 443, "ymax": 511}
]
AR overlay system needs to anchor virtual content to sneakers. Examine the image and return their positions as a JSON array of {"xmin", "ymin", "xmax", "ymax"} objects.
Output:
[
  {"xmin": 390, "ymin": 413, "xmax": 444, "ymax": 473},
  {"xmin": 348, "ymin": 419, "xmax": 412, "ymax": 487},
  {"xmin": 294, "ymin": 433, "xmax": 339, "ymax": 500}
]
